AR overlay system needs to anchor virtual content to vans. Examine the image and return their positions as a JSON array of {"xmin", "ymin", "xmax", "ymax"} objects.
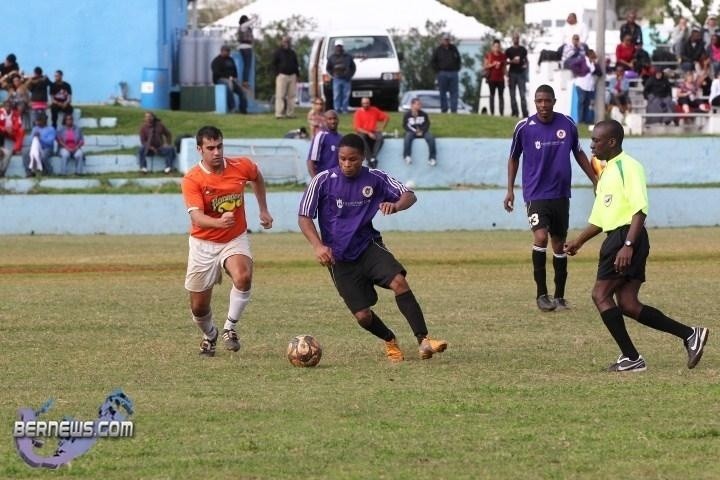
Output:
[{"xmin": 307, "ymin": 28, "xmax": 402, "ymax": 114}]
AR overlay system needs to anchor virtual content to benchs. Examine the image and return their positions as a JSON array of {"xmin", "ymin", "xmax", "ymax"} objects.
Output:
[{"xmin": 606, "ymin": 43, "xmax": 720, "ymax": 136}]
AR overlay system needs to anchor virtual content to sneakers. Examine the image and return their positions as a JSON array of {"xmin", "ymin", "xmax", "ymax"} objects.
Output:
[
  {"xmin": 370, "ymin": 156, "xmax": 436, "ymax": 167},
  {"xmin": 536, "ymin": 294, "xmax": 556, "ymax": 310},
  {"xmin": 608, "ymin": 354, "xmax": 648, "ymax": 373},
  {"xmin": 684, "ymin": 326, "xmax": 709, "ymax": 369},
  {"xmin": 554, "ymin": 298, "xmax": 569, "ymax": 311},
  {"xmin": 384, "ymin": 338, "xmax": 405, "ymax": 363},
  {"xmin": 221, "ymin": 328, "xmax": 241, "ymax": 351},
  {"xmin": 200, "ymin": 328, "xmax": 218, "ymax": 357},
  {"xmin": 418, "ymin": 338, "xmax": 448, "ymax": 360},
  {"xmin": 141, "ymin": 167, "xmax": 172, "ymax": 173}
]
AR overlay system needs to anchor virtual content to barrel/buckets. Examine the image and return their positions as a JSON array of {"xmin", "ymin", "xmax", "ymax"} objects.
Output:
[{"xmin": 140, "ymin": 67, "xmax": 169, "ymax": 109}]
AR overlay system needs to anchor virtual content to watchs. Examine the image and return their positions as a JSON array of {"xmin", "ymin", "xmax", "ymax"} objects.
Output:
[{"xmin": 624, "ymin": 240, "xmax": 634, "ymax": 247}]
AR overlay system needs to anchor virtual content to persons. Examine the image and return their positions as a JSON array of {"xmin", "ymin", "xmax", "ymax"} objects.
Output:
[
  {"xmin": 0, "ymin": 0, "xmax": 720, "ymax": 183},
  {"xmin": 297, "ymin": 132, "xmax": 448, "ymax": 364},
  {"xmin": 181, "ymin": 125, "xmax": 274, "ymax": 358},
  {"xmin": 501, "ymin": 84, "xmax": 597, "ymax": 312},
  {"xmin": 562, "ymin": 117, "xmax": 709, "ymax": 374}
]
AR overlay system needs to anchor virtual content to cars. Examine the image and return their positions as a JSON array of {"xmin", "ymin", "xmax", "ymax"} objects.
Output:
[
  {"xmin": 294, "ymin": 82, "xmax": 312, "ymax": 107},
  {"xmin": 399, "ymin": 90, "xmax": 473, "ymax": 115}
]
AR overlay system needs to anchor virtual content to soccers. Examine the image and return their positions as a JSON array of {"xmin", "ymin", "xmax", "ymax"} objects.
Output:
[{"xmin": 288, "ymin": 335, "xmax": 321, "ymax": 367}]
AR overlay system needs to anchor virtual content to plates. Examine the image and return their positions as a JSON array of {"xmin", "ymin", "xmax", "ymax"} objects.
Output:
[{"xmin": 383, "ymin": 134, "xmax": 393, "ymax": 138}]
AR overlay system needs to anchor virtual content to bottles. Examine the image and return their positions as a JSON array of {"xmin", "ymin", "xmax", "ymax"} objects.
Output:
[
  {"xmin": 394, "ymin": 128, "xmax": 399, "ymax": 138},
  {"xmin": 610, "ymin": 105, "xmax": 643, "ymax": 137}
]
